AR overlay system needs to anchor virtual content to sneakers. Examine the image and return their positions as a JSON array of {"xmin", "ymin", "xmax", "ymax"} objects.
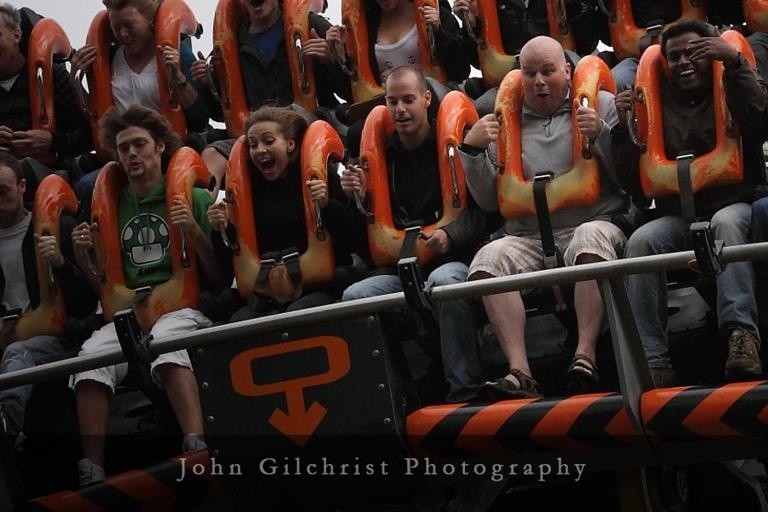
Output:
[
  {"xmin": 181, "ymin": 434, "xmax": 206, "ymax": 453},
  {"xmin": 726, "ymin": 332, "xmax": 762, "ymax": 376},
  {"xmin": 77, "ymin": 458, "xmax": 105, "ymax": 487},
  {"xmin": 649, "ymin": 369, "xmax": 674, "ymax": 388}
]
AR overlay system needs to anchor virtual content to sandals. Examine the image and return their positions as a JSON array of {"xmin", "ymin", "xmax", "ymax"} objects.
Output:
[
  {"xmin": 476, "ymin": 370, "xmax": 544, "ymax": 402},
  {"xmin": 566, "ymin": 355, "xmax": 598, "ymax": 392}
]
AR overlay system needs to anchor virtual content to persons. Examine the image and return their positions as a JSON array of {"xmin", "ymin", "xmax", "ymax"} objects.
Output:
[{"xmin": 0, "ymin": 0, "xmax": 768, "ymax": 487}]
